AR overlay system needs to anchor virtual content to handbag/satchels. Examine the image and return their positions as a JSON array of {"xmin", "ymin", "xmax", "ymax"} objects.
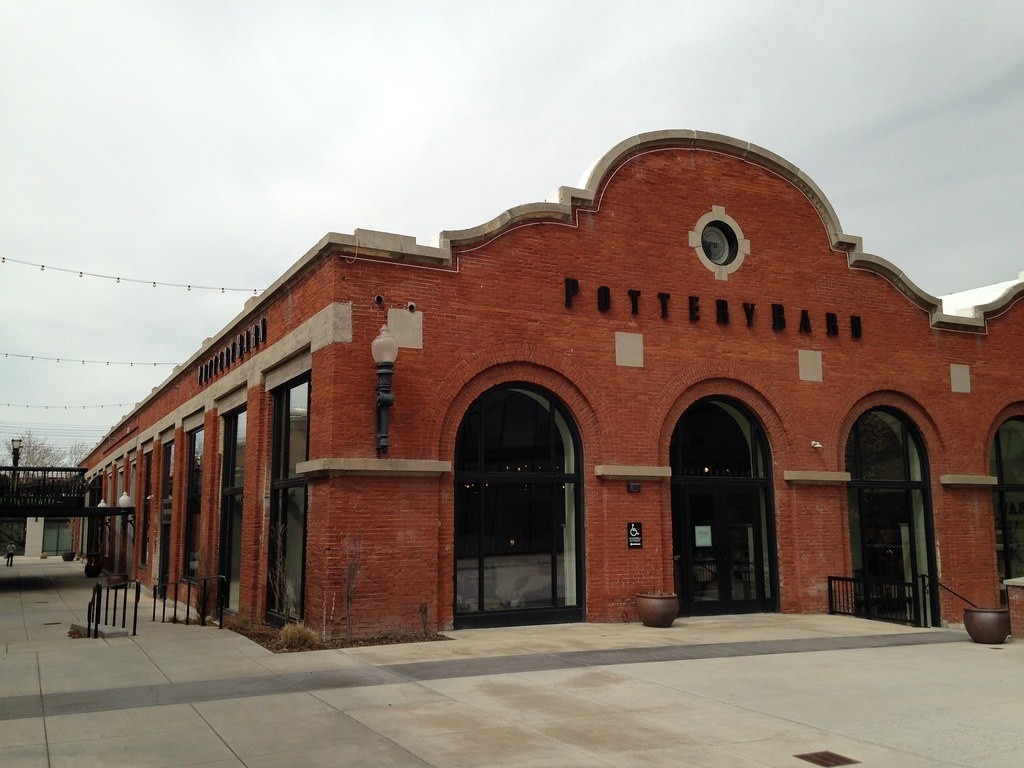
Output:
[{"xmin": 4, "ymin": 553, "xmax": 7, "ymax": 559}]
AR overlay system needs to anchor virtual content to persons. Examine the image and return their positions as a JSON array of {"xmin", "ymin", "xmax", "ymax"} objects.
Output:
[{"xmin": 6, "ymin": 541, "xmax": 17, "ymax": 567}]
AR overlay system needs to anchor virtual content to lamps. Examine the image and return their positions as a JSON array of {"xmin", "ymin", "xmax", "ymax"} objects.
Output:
[
  {"xmin": 118, "ymin": 492, "xmax": 131, "ymax": 507},
  {"xmin": 371, "ymin": 323, "xmax": 399, "ymax": 363},
  {"xmin": 98, "ymin": 499, "xmax": 107, "ymax": 507}
]
